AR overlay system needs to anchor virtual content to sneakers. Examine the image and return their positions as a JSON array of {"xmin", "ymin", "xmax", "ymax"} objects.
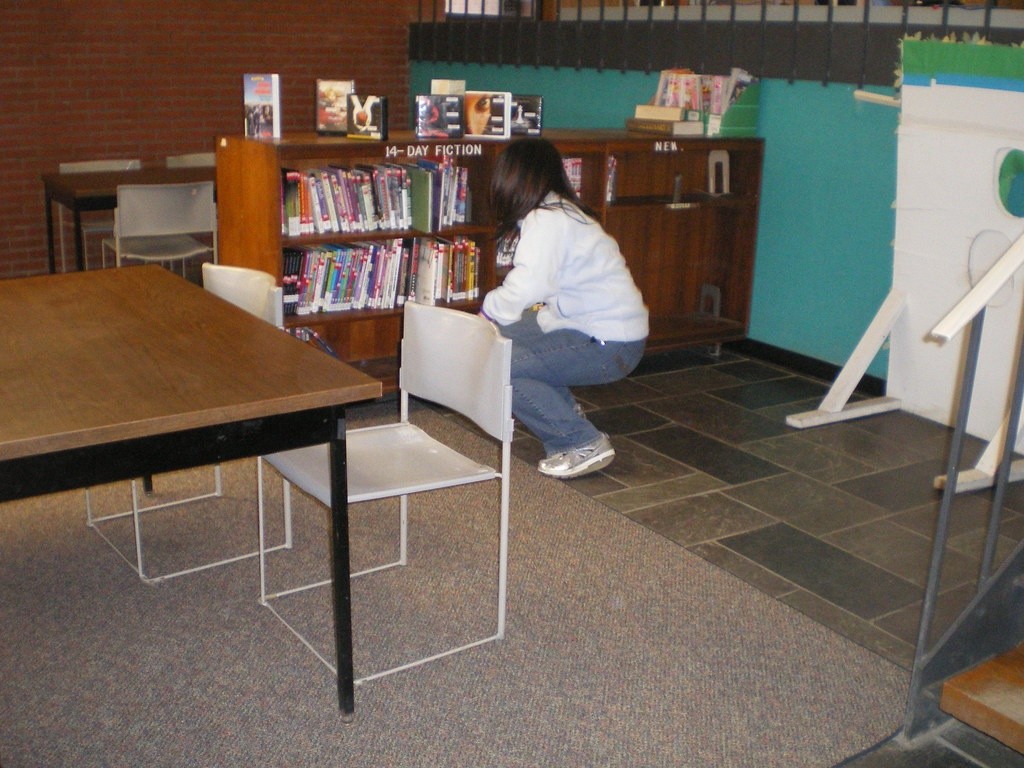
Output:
[
  {"xmin": 573, "ymin": 403, "xmax": 586, "ymax": 417},
  {"xmin": 538, "ymin": 432, "xmax": 616, "ymax": 479}
]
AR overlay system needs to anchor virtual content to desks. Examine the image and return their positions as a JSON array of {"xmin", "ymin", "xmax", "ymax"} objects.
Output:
[
  {"xmin": 0, "ymin": 264, "xmax": 385, "ymax": 724},
  {"xmin": 41, "ymin": 166, "xmax": 217, "ymax": 271}
]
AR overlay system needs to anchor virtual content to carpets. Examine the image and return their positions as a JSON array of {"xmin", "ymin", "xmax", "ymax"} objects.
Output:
[{"xmin": 0, "ymin": 396, "xmax": 914, "ymax": 768}]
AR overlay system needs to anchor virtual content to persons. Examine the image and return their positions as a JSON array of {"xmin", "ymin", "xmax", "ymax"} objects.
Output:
[{"xmin": 481, "ymin": 136, "xmax": 651, "ymax": 480}]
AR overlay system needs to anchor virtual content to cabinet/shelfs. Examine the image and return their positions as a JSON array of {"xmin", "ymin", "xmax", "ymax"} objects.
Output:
[{"xmin": 216, "ymin": 129, "xmax": 765, "ymax": 401}]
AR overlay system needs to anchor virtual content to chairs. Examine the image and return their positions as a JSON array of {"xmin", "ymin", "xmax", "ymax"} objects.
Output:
[
  {"xmin": 57, "ymin": 158, "xmax": 164, "ymax": 268},
  {"xmin": 85, "ymin": 262, "xmax": 294, "ymax": 583},
  {"xmin": 165, "ymin": 153, "xmax": 218, "ymax": 270},
  {"xmin": 258, "ymin": 299, "xmax": 513, "ymax": 685},
  {"xmin": 102, "ymin": 181, "xmax": 218, "ymax": 278}
]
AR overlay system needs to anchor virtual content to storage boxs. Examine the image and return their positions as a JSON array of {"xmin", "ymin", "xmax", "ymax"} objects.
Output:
[
  {"xmin": 346, "ymin": 93, "xmax": 388, "ymax": 140},
  {"xmin": 648, "ymin": 74, "xmax": 763, "ymax": 136},
  {"xmin": 415, "ymin": 93, "xmax": 467, "ymax": 137}
]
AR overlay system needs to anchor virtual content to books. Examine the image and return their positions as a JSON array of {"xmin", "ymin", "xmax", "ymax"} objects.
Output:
[
  {"xmin": 283, "ymin": 235, "xmax": 481, "ymax": 316},
  {"xmin": 243, "ymin": 72, "xmax": 282, "ymax": 143},
  {"xmin": 315, "ymin": 77, "xmax": 355, "ymax": 133},
  {"xmin": 346, "ymin": 93, "xmax": 390, "ymax": 142},
  {"xmin": 626, "ymin": 67, "xmax": 759, "ymax": 136},
  {"xmin": 510, "ymin": 93, "xmax": 543, "ymax": 137},
  {"xmin": 413, "ymin": 93, "xmax": 462, "ymax": 139},
  {"xmin": 278, "ymin": 154, "xmax": 473, "ymax": 237},
  {"xmin": 606, "ymin": 154, "xmax": 618, "ymax": 202},
  {"xmin": 284, "ymin": 326, "xmax": 337, "ymax": 358},
  {"xmin": 561, "ymin": 158, "xmax": 583, "ymax": 198},
  {"xmin": 495, "ymin": 217, "xmax": 520, "ymax": 269},
  {"xmin": 462, "ymin": 90, "xmax": 512, "ymax": 142}
]
